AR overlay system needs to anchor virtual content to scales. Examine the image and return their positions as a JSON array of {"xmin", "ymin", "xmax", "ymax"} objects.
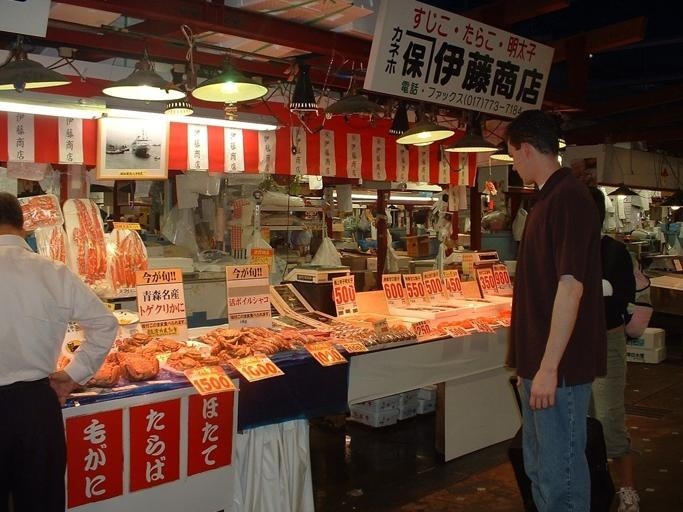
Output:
[
  {"xmin": 283, "ymin": 265, "xmax": 350, "ymax": 283},
  {"xmin": 444, "ymin": 251, "xmax": 499, "ymax": 264}
]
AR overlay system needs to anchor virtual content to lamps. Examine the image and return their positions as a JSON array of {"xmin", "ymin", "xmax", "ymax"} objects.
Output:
[
  {"xmin": 389, "ymin": 96, "xmax": 516, "ymax": 162},
  {"xmin": 607, "ymin": 187, "xmax": 639, "ymax": 197}
]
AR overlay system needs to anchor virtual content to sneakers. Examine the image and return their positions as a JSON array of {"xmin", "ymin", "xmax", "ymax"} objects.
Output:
[{"xmin": 618, "ymin": 486, "xmax": 640, "ymax": 512}]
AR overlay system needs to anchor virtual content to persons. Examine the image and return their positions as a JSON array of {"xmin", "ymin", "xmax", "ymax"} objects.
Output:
[
  {"xmin": 0, "ymin": 192, "xmax": 118, "ymax": 512},
  {"xmin": 15, "ymin": 189, "xmax": 50, "ymax": 254},
  {"xmin": 502, "ymin": 108, "xmax": 609, "ymax": 510},
  {"xmin": 587, "ymin": 184, "xmax": 644, "ymax": 512},
  {"xmin": 290, "ymin": 210, "xmax": 312, "ymax": 256}
]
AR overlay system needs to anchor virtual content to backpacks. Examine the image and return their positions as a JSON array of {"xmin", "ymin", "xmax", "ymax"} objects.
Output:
[{"xmin": 621, "ymin": 257, "xmax": 653, "ymax": 340}]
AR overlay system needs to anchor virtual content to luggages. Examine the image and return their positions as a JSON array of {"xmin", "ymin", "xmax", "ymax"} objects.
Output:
[{"xmin": 509, "ymin": 376, "xmax": 614, "ymax": 512}]
